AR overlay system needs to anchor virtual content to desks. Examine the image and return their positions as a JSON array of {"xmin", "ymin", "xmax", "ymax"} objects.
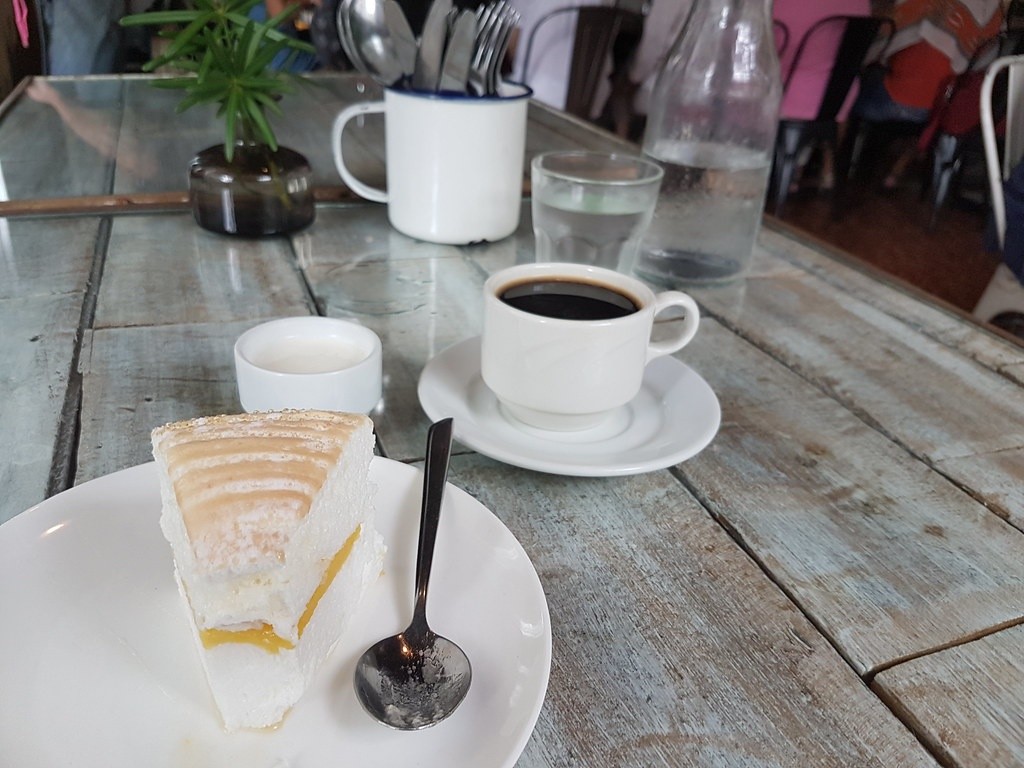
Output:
[{"xmin": 0, "ymin": 73, "xmax": 1024, "ymax": 767}]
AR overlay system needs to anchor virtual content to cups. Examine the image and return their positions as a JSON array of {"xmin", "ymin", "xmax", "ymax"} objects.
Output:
[
  {"xmin": 233, "ymin": 313, "xmax": 383, "ymax": 422},
  {"xmin": 529, "ymin": 149, "xmax": 664, "ymax": 275},
  {"xmin": 331, "ymin": 79, "xmax": 535, "ymax": 247},
  {"xmin": 480, "ymin": 262, "xmax": 700, "ymax": 413}
]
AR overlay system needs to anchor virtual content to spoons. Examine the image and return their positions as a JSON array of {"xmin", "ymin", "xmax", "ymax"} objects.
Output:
[
  {"xmin": 336, "ymin": 0, "xmax": 417, "ymax": 90},
  {"xmin": 352, "ymin": 417, "xmax": 473, "ymax": 733}
]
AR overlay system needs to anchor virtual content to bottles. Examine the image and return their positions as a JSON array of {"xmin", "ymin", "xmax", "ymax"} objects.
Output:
[{"xmin": 626, "ymin": 0, "xmax": 783, "ymax": 284}]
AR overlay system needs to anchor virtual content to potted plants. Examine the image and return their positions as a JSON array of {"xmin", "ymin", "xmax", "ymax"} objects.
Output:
[{"xmin": 119, "ymin": 1, "xmax": 316, "ymax": 235}]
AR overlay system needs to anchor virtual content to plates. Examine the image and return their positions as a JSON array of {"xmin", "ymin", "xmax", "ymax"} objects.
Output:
[
  {"xmin": 414, "ymin": 328, "xmax": 727, "ymax": 479},
  {"xmin": 1, "ymin": 453, "xmax": 554, "ymax": 768}
]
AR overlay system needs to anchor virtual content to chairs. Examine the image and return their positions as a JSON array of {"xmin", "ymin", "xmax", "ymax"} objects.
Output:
[{"xmin": 525, "ymin": 0, "xmax": 1024, "ymax": 335}]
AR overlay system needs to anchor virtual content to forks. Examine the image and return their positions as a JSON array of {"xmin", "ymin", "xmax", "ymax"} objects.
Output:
[{"xmin": 446, "ymin": 1, "xmax": 522, "ymax": 99}]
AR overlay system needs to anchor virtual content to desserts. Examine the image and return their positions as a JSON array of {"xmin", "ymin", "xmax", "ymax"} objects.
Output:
[{"xmin": 151, "ymin": 408, "xmax": 387, "ymax": 730}]
